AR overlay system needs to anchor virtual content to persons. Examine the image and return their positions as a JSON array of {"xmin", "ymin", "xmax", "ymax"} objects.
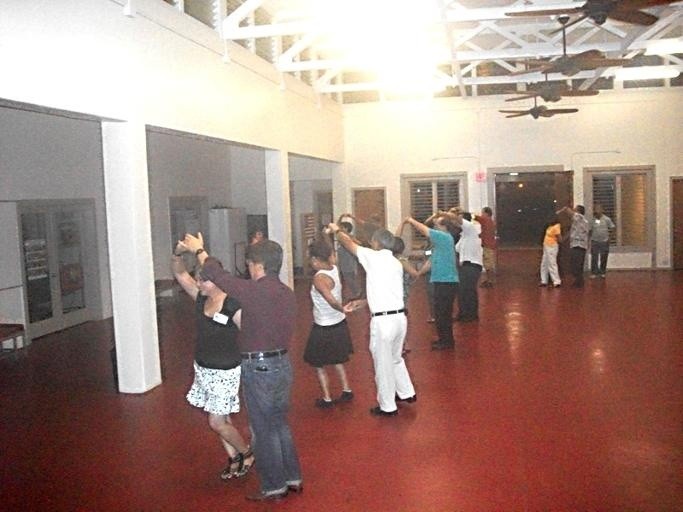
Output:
[
  {"xmin": 172, "ymin": 231, "xmax": 304, "ymax": 501},
  {"xmin": 539, "ymin": 203, "xmax": 616, "ymax": 288},
  {"xmin": 304, "ymin": 207, "xmax": 496, "ymax": 416}
]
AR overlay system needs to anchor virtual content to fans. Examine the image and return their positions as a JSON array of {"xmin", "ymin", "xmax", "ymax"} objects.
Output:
[
  {"xmin": 503, "ymin": 56, "xmax": 598, "ymax": 102},
  {"xmin": 503, "ymin": 0, "xmax": 681, "ymax": 26},
  {"xmin": 499, "ymin": 83, "xmax": 578, "ymax": 118},
  {"xmin": 505, "ymin": 16, "xmax": 661, "ymax": 78}
]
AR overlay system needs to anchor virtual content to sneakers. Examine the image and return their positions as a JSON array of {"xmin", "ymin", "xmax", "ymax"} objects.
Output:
[
  {"xmin": 480, "ymin": 272, "xmax": 607, "ymax": 289},
  {"xmin": 314, "ymin": 397, "xmax": 335, "ymax": 409},
  {"xmin": 429, "ymin": 338, "xmax": 455, "ymax": 350},
  {"xmin": 425, "ymin": 311, "xmax": 478, "ymax": 324},
  {"xmin": 286, "ymin": 483, "xmax": 304, "ymax": 493},
  {"xmin": 339, "ymin": 390, "xmax": 353, "ymax": 401}
]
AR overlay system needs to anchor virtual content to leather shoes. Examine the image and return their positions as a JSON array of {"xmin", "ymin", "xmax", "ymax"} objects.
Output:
[
  {"xmin": 370, "ymin": 406, "xmax": 397, "ymax": 415},
  {"xmin": 394, "ymin": 393, "xmax": 417, "ymax": 403},
  {"xmin": 245, "ymin": 484, "xmax": 288, "ymax": 500}
]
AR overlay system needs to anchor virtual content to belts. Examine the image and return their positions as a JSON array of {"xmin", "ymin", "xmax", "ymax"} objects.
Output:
[
  {"xmin": 371, "ymin": 309, "xmax": 406, "ymax": 316},
  {"xmin": 240, "ymin": 348, "xmax": 289, "ymax": 358}
]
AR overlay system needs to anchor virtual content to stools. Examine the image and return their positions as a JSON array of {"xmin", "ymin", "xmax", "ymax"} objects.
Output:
[
  {"xmin": 0, "ymin": 324, "xmax": 29, "ymax": 369},
  {"xmin": 154, "ymin": 278, "xmax": 175, "ymax": 308}
]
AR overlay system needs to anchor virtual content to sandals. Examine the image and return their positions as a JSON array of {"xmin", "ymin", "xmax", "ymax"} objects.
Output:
[
  {"xmin": 220, "ymin": 453, "xmax": 245, "ymax": 481},
  {"xmin": 236, "ymin": 450, "xmax": 256, "ymax": 478}
]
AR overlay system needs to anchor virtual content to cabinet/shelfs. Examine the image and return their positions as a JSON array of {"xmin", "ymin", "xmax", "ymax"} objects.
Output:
[{"xmin": 208, "ymin": 207, "xmax": 249, "ymax": 279}]
